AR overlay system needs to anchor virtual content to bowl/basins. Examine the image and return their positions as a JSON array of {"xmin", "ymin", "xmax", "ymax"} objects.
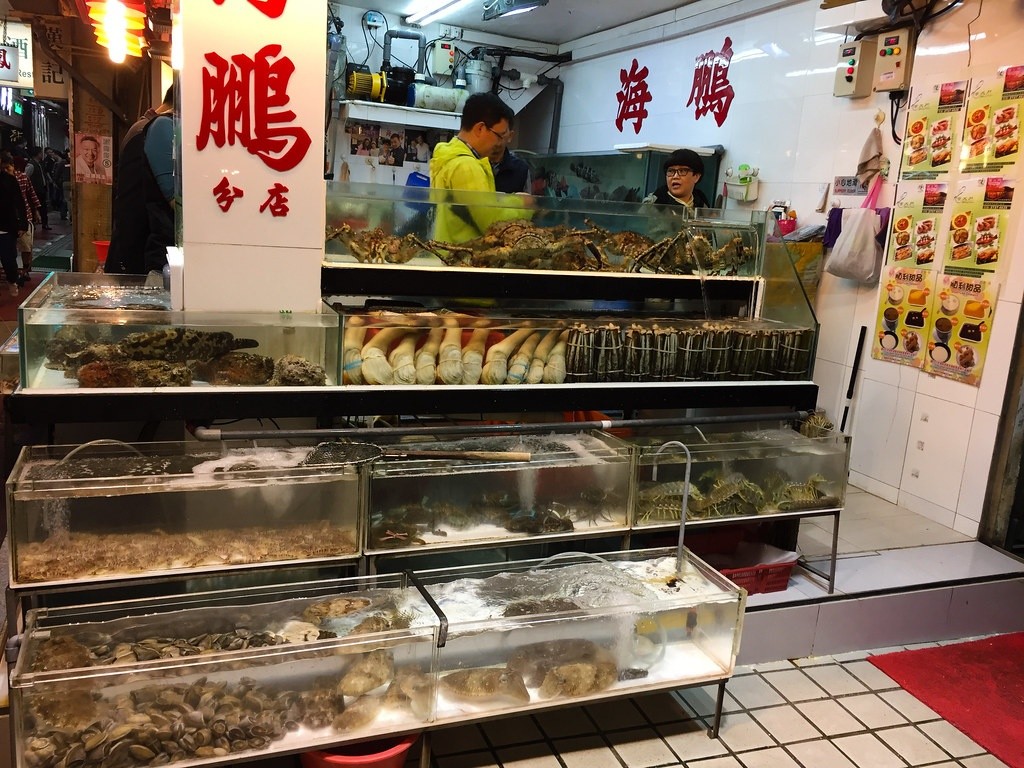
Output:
[{"xmin": 299, "ymin": 734, "xmax": 417, "ymax": 768}]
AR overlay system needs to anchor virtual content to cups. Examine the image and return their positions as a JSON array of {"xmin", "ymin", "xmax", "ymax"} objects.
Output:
[
  {"xmin": 884, "ymin": 307, "xmax": 899, "ymax": 331},
  {"xmin": 942, "ymin": 294, "xmax": 960, "ymax": 311},
  {"xmin": 935, "ymin": 317, "xmax": 953, "ymax": 338},
  {"xmin": 888, "ymin": 285, "xmax": 904, "ymax": 304}
]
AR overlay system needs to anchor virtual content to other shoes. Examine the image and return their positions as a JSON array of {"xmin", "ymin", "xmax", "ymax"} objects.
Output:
[
  {"xmin": 7, "ymin": 280, "xmax": 18, "ymax": 297},
  {"xmin": 42, "ymin": 223, "xmax": 52, "ymax": 230}
]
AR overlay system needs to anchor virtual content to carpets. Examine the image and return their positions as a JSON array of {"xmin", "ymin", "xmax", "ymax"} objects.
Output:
[{"xmin": 868, "ymin": 633, "xmax": 1024, "ymax": 768}]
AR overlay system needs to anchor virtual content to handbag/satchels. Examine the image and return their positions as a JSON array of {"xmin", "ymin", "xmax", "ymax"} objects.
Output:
[{"xmin": 824, "ymin": 174, "xmax": 882, "ymax": 283}]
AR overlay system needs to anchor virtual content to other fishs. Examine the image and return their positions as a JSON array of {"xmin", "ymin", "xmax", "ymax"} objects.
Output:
[
  {"xmin": 302, "ymin": 591, "xmax": 619, "ymax": 733},
  {"xmin": 44, "ymin": 320, "xmax": 326, "ymax": 390}
]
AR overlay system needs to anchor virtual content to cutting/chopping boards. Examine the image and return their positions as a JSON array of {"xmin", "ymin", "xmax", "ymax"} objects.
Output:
[{"xmin": 402, "ymin": 172, "xmax": 430, "ymax": 211}]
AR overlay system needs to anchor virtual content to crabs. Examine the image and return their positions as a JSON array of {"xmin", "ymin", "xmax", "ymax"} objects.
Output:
[
  {"xmin": 369, "ymin": 485, "xmax": 618, "ymax": 550},
  {"xmin": 323, "ymin": 216, "xmax": 756, "ymax": 276}
]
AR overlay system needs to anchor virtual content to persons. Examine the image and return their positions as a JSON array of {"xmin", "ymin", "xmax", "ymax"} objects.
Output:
[
  {"xmin": 12, "ymin": 137, "xmax": 52, "ymax": 230},
  {"xmin": 379, "ymin": 134, "xmax": 404, "ymax": 167},
  {"xmin": 75, "ymin": 136, "xmax": 106, "ymax": 177},
  {"xmin": 135, "ymin": 107, "xmax": 182, "ymax": 276},
  {"xmin": 637, "ymin": 148, "xmax": 719, "ymax": 244},
  {"xmin": 406, "ymin": 135, "xmax": 430, "ymax": 163},
  {"xmin": 488, "ymin": 118, "xmax": 532, "ymax": 193},
  {"xmin": 112, "ymin": 84, "xmax": 173, "ymax": 286},
  {"xmin": 0, "ymin": 150, "xmax": 41, "ymax": 297},
  {"xmin": 356, "ymin": 138, "xmax": 379, "ymax": 157},
  {"xmin": 429, "ymin": 91, "xmax": 538, "ymax": 245},
  {"xmin": 44, "ymin": 146, "xmax": 70, "ymax": 221}
]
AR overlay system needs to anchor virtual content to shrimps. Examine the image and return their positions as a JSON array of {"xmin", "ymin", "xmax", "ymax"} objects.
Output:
[
  {"xmin": 17, "ymin": 519, "xmax": 360, "ymax": 584},
  {"xmin": 630, "ymin": 439, "xmax": 842, "ymax": 519}
]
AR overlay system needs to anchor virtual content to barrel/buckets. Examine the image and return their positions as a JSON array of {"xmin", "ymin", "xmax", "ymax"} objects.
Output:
[
  {"xmin": 777, "ymin": 220, "xmax": 796, "ymax": 235},
  {"xmin": 91, "ymin": 241, "xmax": 111, "ymax": 264}
]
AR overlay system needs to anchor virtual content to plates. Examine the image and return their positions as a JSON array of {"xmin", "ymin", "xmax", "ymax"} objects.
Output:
[
  {"xmin": 963, "ymin": 299, "xmax": 987, "ymax": 319},
  {"xmin": 932, "ymin": 329, "xmax": 952, "ymax": 343},
  {"xmin": 928, "ymin": 342, "xmax": 952, "ymax": 364},
  {"xmin": 903, "ymin": 331, "xmax": 922, "ymax": 354},
  {"xmin": 958, "ymin": 322, "xmax": 984, "ymax": 343},
  {"xmin": 904, "ymin": 309, "xmax": 926, "ymax": 329},
  {"xmin": 907, "ymin": 289, "xmax": 927, "ymax": 306},
  {"xmin": 882, "ymin": 317, "xmax": 899, "ymax": 330},
  {"xmin": 880, "ymin": 331, "xmax": 899, "ymax": 349},
  {"xmin": 956, "ymin": 344, "xmax": 979, "ymax": 369}
]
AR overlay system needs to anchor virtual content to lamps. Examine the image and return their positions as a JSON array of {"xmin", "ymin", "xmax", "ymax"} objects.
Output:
[{"xmin": 480, "ymin": 0, "xmax": 550, "ymax": 22}]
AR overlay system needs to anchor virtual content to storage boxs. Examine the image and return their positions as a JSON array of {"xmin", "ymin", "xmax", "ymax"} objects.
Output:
[
  {"xmin": 7, "ymin": 571, "xmax": 436, "ymax": 768},
  {"xmin": 342, "ymin": 270, "xmax": 784, "ymax": 383},
  {"xmin": 408, "ymin": 544, "xmax": 747, "ymax": 725},
  {"xmin": 522, "ymin": 149, "xmax": 724, "ymax": 207},
  {"xmin": 366, "ymin": 432, "xmax": 632, "ymax": 551},
  {"xmin": 321, "ymin": 180, "xmax": 769, "ymax": 318},
  {"xmin": 634, "ymin": 422, "xmax": 852, "ymax": 594},
  {"xmin": 19, "ymin": 272, "xmax": 340, "ymax": 389},
  {"xmin": 5, "ymin": 439, "xmax": 362, "ymax": 589},
  {"xmin": 710, "ymin": 547, "xmax": 798, "ymax": 592}
]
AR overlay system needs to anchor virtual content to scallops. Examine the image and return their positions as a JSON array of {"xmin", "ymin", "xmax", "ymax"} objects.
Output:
[{"xmin": 23, "ymin": 620, "xmax": 315, "ymax": 767}]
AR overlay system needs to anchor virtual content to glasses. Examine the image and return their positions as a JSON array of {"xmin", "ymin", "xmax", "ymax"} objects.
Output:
[
  {"xmin": 666, "ymin": 167, "xmax": 694, "ymax": 177},
  {"xmin": 486, "ymin": 126, "xmax": 506, "ymax": 142}
]
